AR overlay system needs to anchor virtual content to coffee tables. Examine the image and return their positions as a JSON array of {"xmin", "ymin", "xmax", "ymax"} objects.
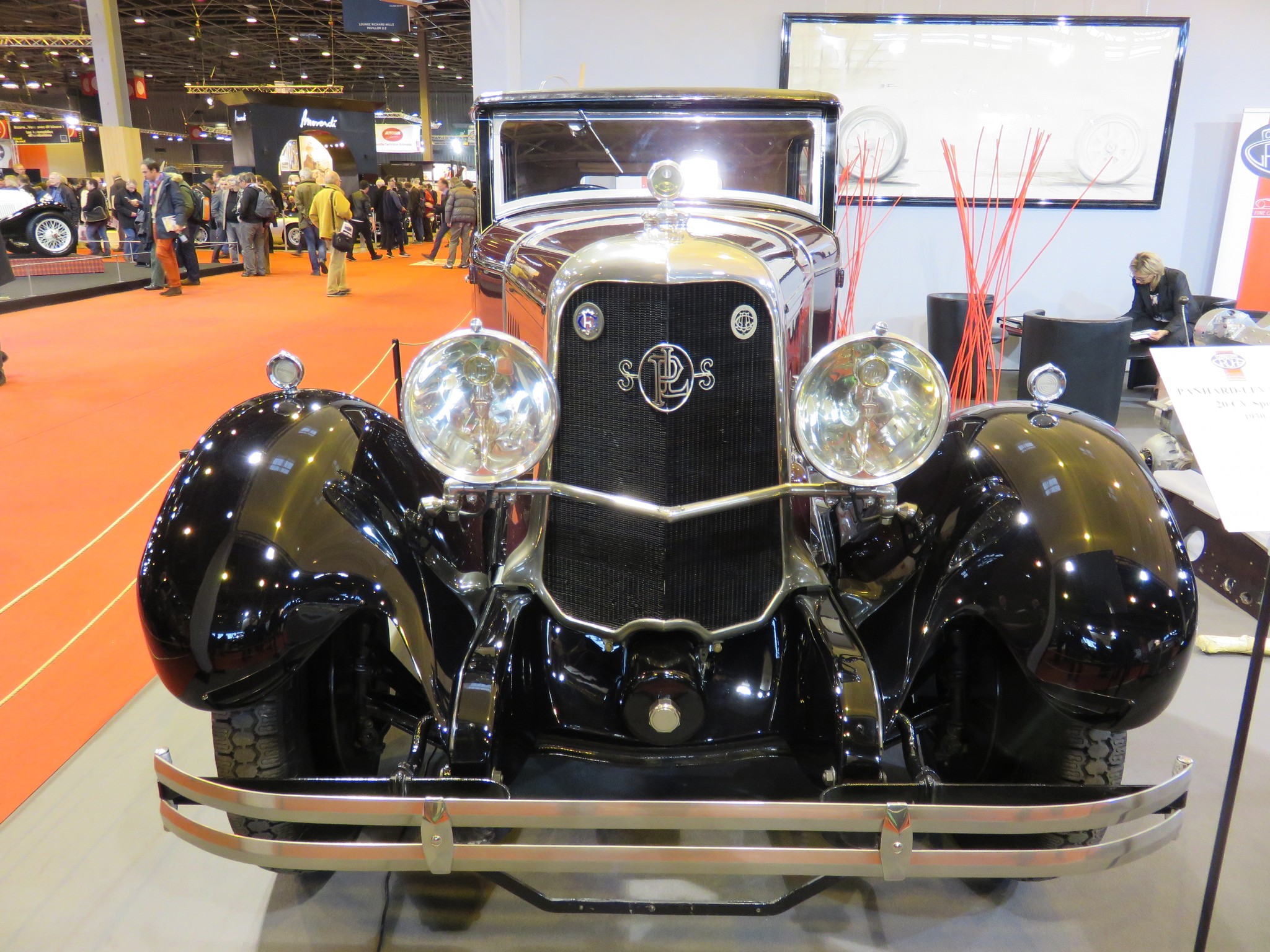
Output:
[{"xmin": 1008, "ymin": 334, "xmax": 1151, "ymax": 359}]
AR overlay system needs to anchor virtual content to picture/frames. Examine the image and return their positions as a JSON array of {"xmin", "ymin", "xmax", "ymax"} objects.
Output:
[{"xmin": 778, "ymin": 13, "xmax": 1191, "ymax": 210}]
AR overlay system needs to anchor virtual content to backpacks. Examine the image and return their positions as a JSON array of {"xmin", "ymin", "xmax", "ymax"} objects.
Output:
[
  {"xmin": 180, "ymin": 184, "xmax": 211, "ymax": 223},
  {"xmin": 247, "ymin": 186, "xmax": 275, "ymax": 218},
  {"xmin": 256, "ymin": 186, "xmax": 279, "ymax": 216}
]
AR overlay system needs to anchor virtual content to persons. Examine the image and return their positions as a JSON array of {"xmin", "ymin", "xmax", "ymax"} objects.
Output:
[
  {"xmin": 0, "ymin": 229, "xmax": 17, "ymax": 385},
  {"xmin": 1113, "ymin": 252, "xmax": 1202, "ymax": 401},
  {"xmin": 308, "ymin": 170, "xmax": 353, "ymax": 297},
  {"xmin": 0, "ymin": 164, "xmax": 477, "ymax": 288},
  {"xmin": 141, "ymin": 158, "xmax": 187, "ymax": 296}
]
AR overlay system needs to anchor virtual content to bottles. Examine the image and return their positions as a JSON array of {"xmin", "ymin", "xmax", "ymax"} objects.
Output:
[{"xmin": 178, "ymin": 233, "xmax": 189, "ymax": 244}]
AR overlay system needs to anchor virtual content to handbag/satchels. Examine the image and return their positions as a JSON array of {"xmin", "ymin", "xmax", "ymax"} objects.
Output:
[
  {"xmin": 405, "ymin": 218, "xmax": 410, "ymax": 228},
  {"xmin": 333, "ymin": 233, "xmax": 353, "ymax": 252},
  {"xmin": 83, "ymin": 206, "xmax": 107, "ymax": 222}
]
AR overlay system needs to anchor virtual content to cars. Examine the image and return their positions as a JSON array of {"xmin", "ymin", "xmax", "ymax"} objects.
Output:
[
  {"xmin": 0, "ymin": 180, "xmax": 80, "ymax": 258},
  {"xmin": 137, "ymin": 83, "xmax": 1205, "ymax": 880},
  {"xmin": 269, "ymin": 211, "xmax": 303, "ymax": 249},
  {"xmin": 194, "ymin": 222, "xmax": 213, "ymax": 250}
]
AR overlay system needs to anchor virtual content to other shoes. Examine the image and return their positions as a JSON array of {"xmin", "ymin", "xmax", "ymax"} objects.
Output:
[
  {"xmin": 89, "ymin": 249, "xmax": 111, "ymax": 258},
  {"xmin": 340, "ymin": 289, "xmax": 350, "ymax": 293},
  {"xmin": 254, "ymin": 273, "xmax": 266, "ymax": 276},
  {"xmin": 180, "ymin": 279, "xmax": 200, "ymax": 285},
  {"xmin": 159, "ymin": 287, "xmax": 182, "ymax": 296},
  {"xmin": 327, "ymin": 291, "xmax": 347, "ymax": 296},
  {"xmin": 346, "ymin": 255, "xmax": 356, "ymax": 261},
  {"xmin": 113, "ymin": 247, "xmax": 125, "ymax": 252},
  {"xmin": 458, "ymin": 264, "xmax": 467, "ymax": 268},
  {"xmin": 241, "ymin": 270, "xmax": 257, "ymax": 276},
  {"xmin": 290, "ymin": 253, "xmax": 302, "ymax": 257},
  {"xmin": 311, "ymin": 262, "xmax": 328, "ymax": 276},
  {"xmin": 218, "ymin": 253, "xmax": 230, "ymax": 259},
  {"xmin": 372, "ymin": 254, "xmax": 382, "ymax": 261},
  {"xmin": 143, "ymin": 284, "xmax": 163, "ymax": 290},
  {"xmin": 124, "ymin": 256, "xmax": 129, "ymax": 261},
  {"xmin": 443, "ymin": 265, "xmax": 452, "ymax": 269},
  {"xmin": 270, "ymin": 250, "xmax": 274, "ymax": 253},
  {"xmin": 379, "ymin": 239, "xmax": 468, "ymax": 259}
]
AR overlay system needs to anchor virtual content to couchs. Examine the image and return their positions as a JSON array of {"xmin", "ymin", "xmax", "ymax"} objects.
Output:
[
  {"xmin": 1126, "ymin": 295, "xmax": 1237, "ymax": 390},
  {"xmin": 1017, "ymin": 309, "xmax": 1134, "ymax": 427},
  {"xmin": 926, "ymin": 293, "xmax": 1009, "ymax": 399}
]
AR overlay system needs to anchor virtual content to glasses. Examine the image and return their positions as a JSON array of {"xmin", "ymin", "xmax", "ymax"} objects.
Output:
[
  {"xmin": 389, "ymin": 180, "xmax": 396, "ymax": 182},
  {"xmin": 1129, "ymin": 271, "xmax": 1152, "ymax": 279}
]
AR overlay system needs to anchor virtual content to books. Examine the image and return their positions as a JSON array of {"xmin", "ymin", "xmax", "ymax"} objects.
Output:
[{"xmin": 1130, "ymin": 329, "xmax": 1156, "ymax": 341}]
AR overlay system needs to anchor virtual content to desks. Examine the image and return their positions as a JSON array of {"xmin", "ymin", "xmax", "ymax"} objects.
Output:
[{"xmin": 1149, "ymin": 345, "xmax": 1270, "ymax": 532}]
